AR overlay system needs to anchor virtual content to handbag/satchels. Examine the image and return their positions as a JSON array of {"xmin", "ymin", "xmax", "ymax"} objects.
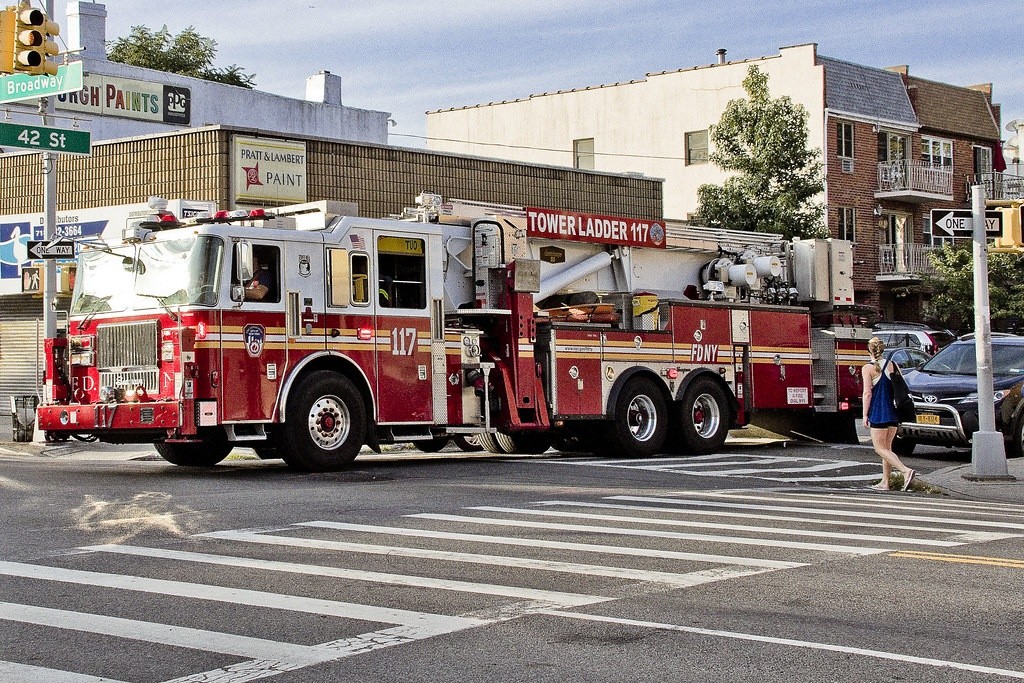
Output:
[{"xmin": 889, "ymin": 361, "xmax": 909, "ymax": 397}]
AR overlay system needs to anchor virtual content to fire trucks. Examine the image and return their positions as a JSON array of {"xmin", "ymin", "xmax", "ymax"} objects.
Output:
[{"xmin": 34, "ymin": 188, "xmax": 882, "ymax": 480}]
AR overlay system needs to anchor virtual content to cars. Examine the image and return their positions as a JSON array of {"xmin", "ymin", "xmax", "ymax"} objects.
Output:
[{"xmin": 880, "ymin": 347, "xmax": 953, "ymax": 377}]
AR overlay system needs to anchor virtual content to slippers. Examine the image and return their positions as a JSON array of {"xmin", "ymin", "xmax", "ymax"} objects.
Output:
[
  {"xmin": 901, "ymin": 470, "xmax": 915, "ymax": 492},
  {"xmin": 871, "ymin": 484, "xmax": 891, "ymax": 491}
]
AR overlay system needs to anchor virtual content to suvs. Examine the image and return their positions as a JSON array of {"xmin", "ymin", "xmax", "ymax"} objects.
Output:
[
  {"xmin": 890, "ymin": 331, "xmax": 1024, "ymax": 457},
  {"xmin": 870, "ymin": 320, "xmax": 957, "ymax": 358}
]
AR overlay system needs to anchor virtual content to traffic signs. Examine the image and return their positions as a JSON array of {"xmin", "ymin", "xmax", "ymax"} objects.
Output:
[
  {"xmin": 27, "ymin": 240, "xmax": 76, "ymax": 259},
  {"xmin": 930, "ymin": 209, "xmax": 1004, "ymax": 239}
]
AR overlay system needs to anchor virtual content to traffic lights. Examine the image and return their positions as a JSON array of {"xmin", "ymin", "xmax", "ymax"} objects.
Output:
[
  {"xmin": 60, "ymin": 267, "xmax": 78, "ymax": 293},
  {"xmin": 41, "ymin": 12, "xmax": 59, "ymax": 77},
  {"xmin": 22, "ymin": 264, "xmax": 43, "ymax": 296},
  {"xmin": 11, "ymin": 5, "xmax": 46, "ymax": 75}
]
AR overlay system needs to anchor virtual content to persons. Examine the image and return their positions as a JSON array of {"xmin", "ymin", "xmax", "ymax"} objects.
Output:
[
  {"xmin": 230, "ymin": 248, "xmax": 277, "ymax": 303},
  {"xmin": 862, "ymin": 336, "xmax": 916, "ymax": 492}
]
